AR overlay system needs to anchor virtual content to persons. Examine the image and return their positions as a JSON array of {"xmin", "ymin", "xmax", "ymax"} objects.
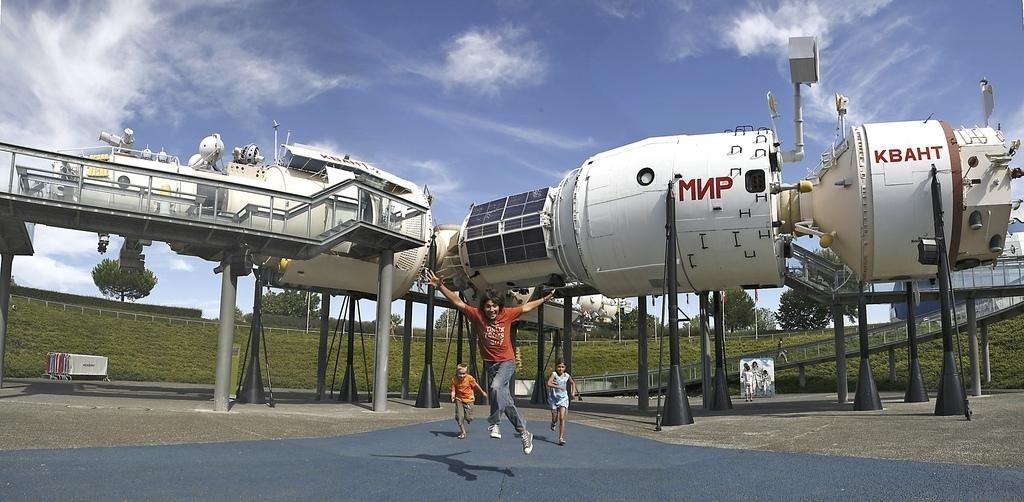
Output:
[
  {"xmin": 751, "ymin": 361, "xmax": 762, "ymax": 395},
  {"xmin": 761, "ymin": 369, "xmax": 771, "ymax": 396},
  {"xmin": 548, "ymin": 359, "xmax": 575, "ymax": 448},
  {"xmin": 741, "ymin": 362, "xmax": 748, "ymax": 379},
  {"xmin": 744, "ymin": 365, "xmax": 752, "ymax": 402},
  {"xmin": 776, "ymin": 338, "xmax": 789, "ymax": 364},
  {"xmin": 448, "ymin": 362, "xmax": 489, "ymax": 439},
  {"xmin": 420, "ymin": 269, "xmax": 555, "ymax": 455}
]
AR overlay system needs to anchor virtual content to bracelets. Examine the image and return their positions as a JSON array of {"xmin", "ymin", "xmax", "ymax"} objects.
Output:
[{"xmin": 542, "ymin": 296, "xmax": 547, "ymax": 302}]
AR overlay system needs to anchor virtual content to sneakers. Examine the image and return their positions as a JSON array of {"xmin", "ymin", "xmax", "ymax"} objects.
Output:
[
  {"xmin": 550, "ymin": 422, "xmax": 566, "ymax": 447},
  {"xmin": 520, "ymin": 429, "xmax": 534, "ymax": 455},
  {"xmin": 456, "ymin": 418, "xmax": 473, "ymax": 440},
  {"xmin": 487, "ymin": 423, "xmax": 501, "ymax": 439}
]
